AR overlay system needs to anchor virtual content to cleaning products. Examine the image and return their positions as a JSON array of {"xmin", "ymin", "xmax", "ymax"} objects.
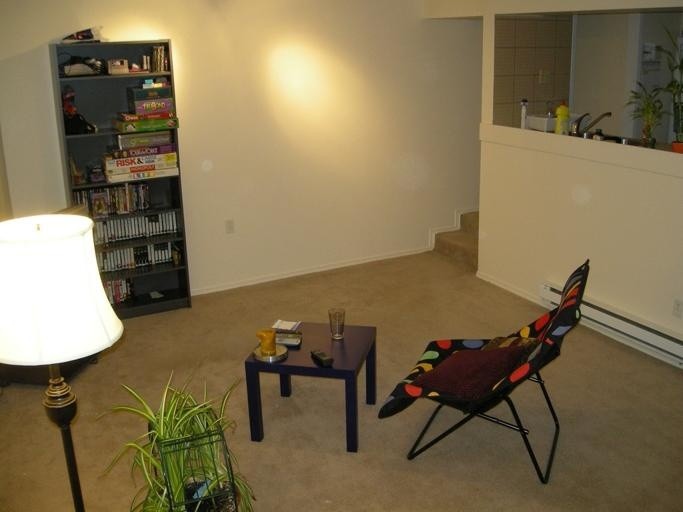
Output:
[{"xmin": 519, "ymin": 99, "xmax": 528, "ymax": 129}]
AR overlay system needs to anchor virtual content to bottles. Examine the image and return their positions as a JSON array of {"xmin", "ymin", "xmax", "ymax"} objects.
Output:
[
  {"xmin": 556, "ymin": 101, "xmax": 570, "ymax": 135},
  {"xmin": 594, "ymin": 129, "xmax": 604, "ymax": 141}
]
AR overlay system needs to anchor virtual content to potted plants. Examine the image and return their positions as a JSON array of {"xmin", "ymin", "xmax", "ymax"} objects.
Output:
[
  {"xmin": 621, "ymin": 22, "xmax": 683, "ymax": 154},
  {"xmin": 93, "ymin": 356, "xmax": 258, "ymax": 511}
]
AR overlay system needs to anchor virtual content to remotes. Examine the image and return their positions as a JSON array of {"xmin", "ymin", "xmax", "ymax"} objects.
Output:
[{"xmin": 310, "ymin": 348, "xmax": 334, "ymax": 367}]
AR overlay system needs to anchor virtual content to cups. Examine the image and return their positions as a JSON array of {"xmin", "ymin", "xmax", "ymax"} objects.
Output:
[{"xmin": 328, "ymin": 308, "xmax": 345, "ymax": 340}]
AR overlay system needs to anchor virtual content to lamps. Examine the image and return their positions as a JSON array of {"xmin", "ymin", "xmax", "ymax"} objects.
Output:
[{"xmin": 1, "ymin": 214, "xmax": 124, "ymax": 511}]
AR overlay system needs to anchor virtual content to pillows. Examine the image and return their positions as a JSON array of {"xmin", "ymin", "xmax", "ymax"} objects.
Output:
[{"xmin": 415, "ymin": 336, "xmax": 539, "ymax": 399}]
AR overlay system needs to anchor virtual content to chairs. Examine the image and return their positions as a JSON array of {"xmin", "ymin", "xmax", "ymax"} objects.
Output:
[{"xmin": 377, "ymin": 258, "xmax": 591, "ymax": 484}]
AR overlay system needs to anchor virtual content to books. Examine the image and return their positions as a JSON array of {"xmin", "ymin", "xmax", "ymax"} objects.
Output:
[{"xmin": 58, "ymin": 44, "xmax": 183, "ymax": 305}]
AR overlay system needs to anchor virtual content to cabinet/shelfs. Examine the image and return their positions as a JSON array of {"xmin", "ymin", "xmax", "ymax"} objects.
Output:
[{"xmin": 48, "ymin": 39, "xmax": 191, "ymax": 320}]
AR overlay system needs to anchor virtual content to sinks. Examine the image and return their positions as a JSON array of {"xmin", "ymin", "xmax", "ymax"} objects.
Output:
[
  {"xmin": 571, "ymin": 112, "xmax": 612, "ymax": 135},
  {"xmin": 609, "ymin": 136, "xmax": 643, "ymax": 146}
]
